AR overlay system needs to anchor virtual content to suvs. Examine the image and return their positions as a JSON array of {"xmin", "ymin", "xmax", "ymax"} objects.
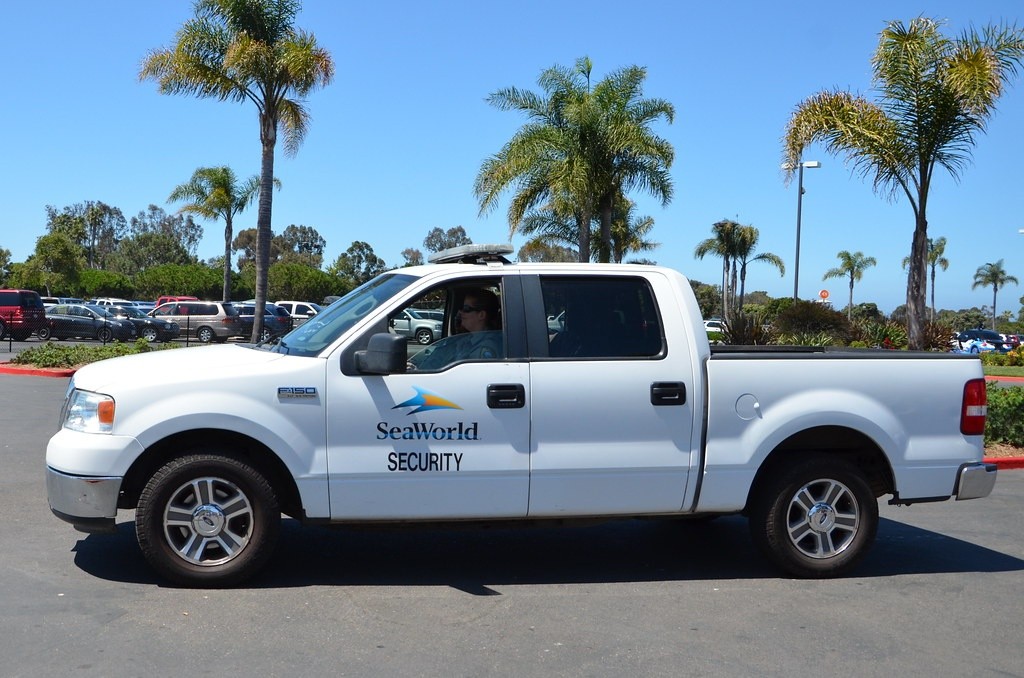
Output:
[
  {"xmin": 393, "ymin": 307, "xmax": 445, "ymax": 345},
  {"xmin": 40, "ymin": 295, "xmax": 202, "ymax": 315},
  {"xmin": 231, "ymin": 303, "xmax": 293, "ymax": 343}
]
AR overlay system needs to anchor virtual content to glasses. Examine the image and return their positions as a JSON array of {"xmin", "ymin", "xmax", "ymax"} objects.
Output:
[{"xmin": 460, "ymin": 305, "xmax": 480, "ymax": 314}]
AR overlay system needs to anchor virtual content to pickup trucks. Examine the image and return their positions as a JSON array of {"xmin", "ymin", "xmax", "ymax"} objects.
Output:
[{"xmin": 44, "ymin": 244, "xmax": 997, "ymax": 588}]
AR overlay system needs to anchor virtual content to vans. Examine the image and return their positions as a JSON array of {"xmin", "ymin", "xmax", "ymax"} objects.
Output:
[
  {"xmin": 147, "ymin": 301, "xmax": 242, "ymax": 343},
  {"xmin": 275, "ymin": 300, "xmax": 324, "ymax": 329},
  {"xmin": 0, "ymin": 288, "xmax": 46, "ymax": 341}
]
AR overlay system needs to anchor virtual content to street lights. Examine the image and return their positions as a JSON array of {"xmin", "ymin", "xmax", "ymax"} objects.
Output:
[{"xmin": 781, "ymin": 160, "xmax": 821, "ymax": 307}]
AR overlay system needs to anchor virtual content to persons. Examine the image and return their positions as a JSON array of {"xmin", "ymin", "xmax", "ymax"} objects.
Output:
[{"xmin": 407, "ymin": 289, "xmax": 499, "ymax": 370}]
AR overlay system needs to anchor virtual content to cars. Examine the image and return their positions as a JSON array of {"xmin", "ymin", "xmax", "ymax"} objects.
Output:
[
  {"xmin": 545, "ymin": 308, "xmax": 566, "ymax": 334},
  {"xmin": 704, "ymin": 319, "xmax": 729, "ymax": 333},
  {"xmin": 96, "ymin": 304, "xmax": 180, "ymax": 342},
  {"xmin": 953, "ymin": 329, "xmax": 1024, "ymax": 354},
  {"xmin": 34, "ymin": 304, "xmax": 138, "ymax": 343}
]
